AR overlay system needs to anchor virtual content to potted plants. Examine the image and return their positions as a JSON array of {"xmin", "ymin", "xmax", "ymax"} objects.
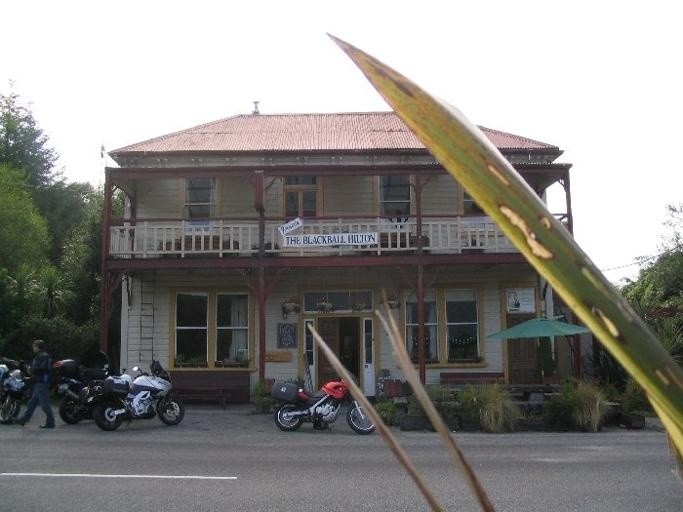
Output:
[{"xmin": 374, "ymin": 378, "xmax": 644, "ymax": 434}]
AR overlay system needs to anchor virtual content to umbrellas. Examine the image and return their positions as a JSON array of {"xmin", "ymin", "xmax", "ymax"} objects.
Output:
[{"xmin": 483, "ymin": 316, "xmax": 591, "ymax": 383}]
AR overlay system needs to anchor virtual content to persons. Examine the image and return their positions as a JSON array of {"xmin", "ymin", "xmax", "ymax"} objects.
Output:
[{"xmin": 8, "ymin": 339, "xmax": 55, "ymax": 428}]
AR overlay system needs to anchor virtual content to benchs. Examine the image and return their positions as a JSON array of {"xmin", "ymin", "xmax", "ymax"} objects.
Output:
[
  {"xmin": 157, "ymin": 232, "xmax": 486, "ymax": 258},
  {"xmin": 170, "ymin": 375, "xmax": 231, "ymax": 410}
]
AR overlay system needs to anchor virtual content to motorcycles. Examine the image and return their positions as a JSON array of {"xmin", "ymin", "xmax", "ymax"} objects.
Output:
[
  {"xmin": 54, "ymin": 352, "xmax": 125, "ymax": 425},
  {"xmin": 81, "ymin": 361, "xmax": 186, "ymax": 430},
  {"xmin": 269, "ymin": 368, "xmax": 377, "ymax": 435},
  {"xmin": 0, "ymin": 357, "xmax": 35, "ymax": 425}
]
